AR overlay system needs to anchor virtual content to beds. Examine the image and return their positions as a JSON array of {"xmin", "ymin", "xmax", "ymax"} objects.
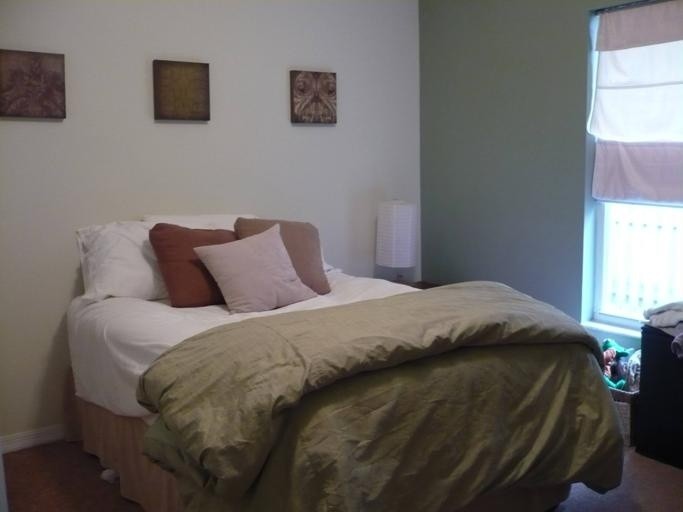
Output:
[{"xmin": 65, "ymin": 213, "xmax": 623, "ymax": 512}]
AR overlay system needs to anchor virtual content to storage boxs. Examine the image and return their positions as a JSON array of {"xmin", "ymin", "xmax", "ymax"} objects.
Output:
[{"xmin": 608, "ymin": 387, "xmax": 639, "ymax": 448}]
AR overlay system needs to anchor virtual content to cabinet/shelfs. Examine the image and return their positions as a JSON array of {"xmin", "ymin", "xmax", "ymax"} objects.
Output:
[{"xmin": 635, "ymin": 325, "xmax": 683, "ymax": 460}]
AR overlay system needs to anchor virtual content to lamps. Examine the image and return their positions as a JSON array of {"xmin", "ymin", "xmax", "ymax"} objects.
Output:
[{"xmin": 376, "ymin": 200, "xmax": 418, "ymax": 271}]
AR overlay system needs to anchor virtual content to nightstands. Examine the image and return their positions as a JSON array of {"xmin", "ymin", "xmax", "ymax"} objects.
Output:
[{"xmin": 401, "ymin": 280, "xmax": 444, "ymax": 289}]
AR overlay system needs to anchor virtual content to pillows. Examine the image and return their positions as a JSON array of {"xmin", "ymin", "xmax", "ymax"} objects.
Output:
[
  {"xmin": 148, "ymin": 223, "xmax": 240, "ymax": 307},
  {"xmin": 192, "ymin": 222, "xmax": 316, "ymax": 316},
  {"xmin": 235, "ymin": 217, "xmax": 331, "ymax": 296},
  {"xmin": 73, "ymin": 221, "xmax": 236, "ymax": 300}
]
